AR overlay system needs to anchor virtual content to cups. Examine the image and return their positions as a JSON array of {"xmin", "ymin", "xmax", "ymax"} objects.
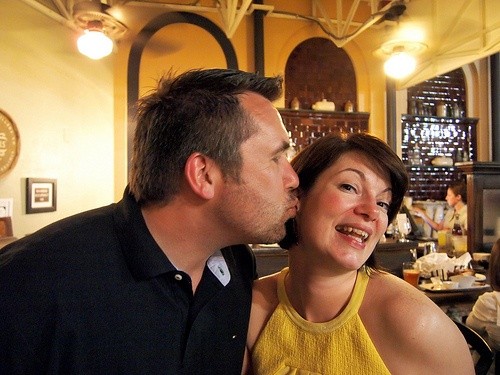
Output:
[
  {"xmin": 452, "ymin": 235, "xmax": 467, "ymax": 258},
  {"xmin": 437, "ymin": 230, "xmax": 448, "ymax": 249},
  {"xmin": 403, "ymin": 262, "xmax": 420, "ymax": 287}
]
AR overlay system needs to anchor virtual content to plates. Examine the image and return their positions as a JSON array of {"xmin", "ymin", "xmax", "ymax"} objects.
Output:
[{"xmin": 473, "ymin": 273, "xmax": 488, "ymax": 282}]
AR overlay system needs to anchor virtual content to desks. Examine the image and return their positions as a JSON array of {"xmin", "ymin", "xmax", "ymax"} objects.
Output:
[{"xmin": 249, "ymin": 240, "xmax": 418, "ymax": 280}]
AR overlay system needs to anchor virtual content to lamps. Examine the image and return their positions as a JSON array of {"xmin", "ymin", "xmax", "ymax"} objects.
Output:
[
  {"xmin": 376, "ymin": 5, "xmax": 428, "ymax": 81},
  {"xmin": 75, "ymin": 0, "xmax": 128, "ymax": 61}
]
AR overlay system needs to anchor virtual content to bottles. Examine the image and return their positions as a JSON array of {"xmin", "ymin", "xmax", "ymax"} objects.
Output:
[{"xmin": 452, "ymin": 215, "xmax": 463, "ymax": 235}]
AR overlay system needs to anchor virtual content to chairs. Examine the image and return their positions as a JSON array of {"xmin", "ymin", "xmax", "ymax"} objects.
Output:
[{"xmin": 455, "ymin": 320, "xmax": 495, "ymax": 375}]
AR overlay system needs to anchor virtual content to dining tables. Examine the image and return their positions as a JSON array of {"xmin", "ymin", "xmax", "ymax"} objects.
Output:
[{"xmin": 420, "ymin": 279, "xmax": 490, "ymax": 302}]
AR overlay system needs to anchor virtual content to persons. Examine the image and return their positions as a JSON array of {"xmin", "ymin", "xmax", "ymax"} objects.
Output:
[
  {"xmin": 0, "ymin": 65, "xmax": 300, "ymax": 375},
  {"xmin": 414, "ymin": 181, "xmax": 468, "ymax": 237},
  {"xmin": 241, "ymin": 131, "xmax": 476, "ymax": 375},
  {"xmin": 466, "ymin": 237, "xmax": 500, "ymax": 375}
]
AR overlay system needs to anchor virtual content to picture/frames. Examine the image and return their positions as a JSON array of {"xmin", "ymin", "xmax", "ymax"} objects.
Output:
[{"xmin": 26, "ymin": 177, "xmax": 57, "ymax": 213}]
